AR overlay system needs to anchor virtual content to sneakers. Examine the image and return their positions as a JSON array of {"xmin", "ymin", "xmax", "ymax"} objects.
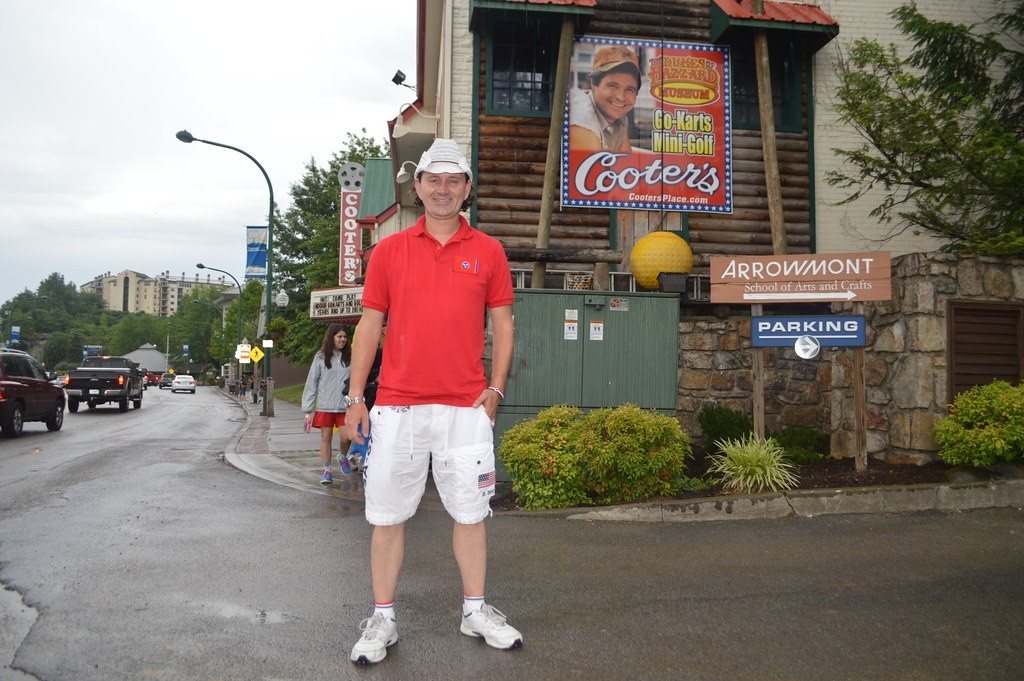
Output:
[
  {"xmin": 336, "ymin": 454, "xmax": 351, "ymax": 475},
  {"xmin": 320, "ymin": 463, "xmax": 332, "ymax": 483},
  {"xmin": 459, "ymin": 603, "xmax": 523, "ymax": 649},
  {"xmin": 349, "ymin": 612, "xmax": 399, "ymax": 664}
]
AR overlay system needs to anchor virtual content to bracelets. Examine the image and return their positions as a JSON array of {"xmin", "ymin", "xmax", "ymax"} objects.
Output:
[{"xmin": 488, "ymin": 386, "xmax": 504, "ymax": 400}]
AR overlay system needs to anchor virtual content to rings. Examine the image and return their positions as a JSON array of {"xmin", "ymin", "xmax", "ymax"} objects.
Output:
[{"xmin": 490, "ymin": 421, "xmax": 495, "ymax": 427}]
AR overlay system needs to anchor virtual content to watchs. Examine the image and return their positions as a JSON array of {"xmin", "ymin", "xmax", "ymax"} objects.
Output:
[{"xmin": 344, "ymin": 395, "xmax": 365, "ymax": 408}]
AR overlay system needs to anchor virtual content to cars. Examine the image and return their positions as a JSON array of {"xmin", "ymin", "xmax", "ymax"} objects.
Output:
[
  {"xmin": 159, "ymin": 373, "xmax": 176, "ymax": 389},
  {"xmin": 171, "ymin": 374, "xmax": 196, "ymax": 394},
  {"xmin": 138, "ymin": 368, "xmax": 161, "ymax": 390}
]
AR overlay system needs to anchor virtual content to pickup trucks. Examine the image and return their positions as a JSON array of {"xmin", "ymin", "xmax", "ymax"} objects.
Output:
[{"xmin": 65, "ymin": 355, "xmax": 148, "ymax": 413}]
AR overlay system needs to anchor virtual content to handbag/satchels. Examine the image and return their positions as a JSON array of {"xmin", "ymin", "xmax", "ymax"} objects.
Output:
[{"xmin": 304, "ymin": 413, "xmax": 311, "ymax": 434}]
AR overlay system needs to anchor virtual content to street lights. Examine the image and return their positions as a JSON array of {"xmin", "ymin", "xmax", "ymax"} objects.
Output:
[
  {"xmin": 197, "ymin": 263, "xmax": 240, "ymax": 395},
  {"xmin": 175, "ymin": 129, "xmax": 271, "ymax": 416}
]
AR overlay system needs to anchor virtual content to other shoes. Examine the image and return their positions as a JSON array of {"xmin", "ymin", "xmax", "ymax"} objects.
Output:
[
  {"xmin": 357, "ymin": 458, "xmax": 364, "ymax": 473},
  {"xmin": 347, "ymin": 458, "xmax": 357, "ymax": 471}
]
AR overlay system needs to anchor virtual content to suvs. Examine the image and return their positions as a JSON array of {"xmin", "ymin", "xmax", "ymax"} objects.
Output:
[{"xmin": 0, "ymin": 348, "xmax": 66, "ymax": 440}]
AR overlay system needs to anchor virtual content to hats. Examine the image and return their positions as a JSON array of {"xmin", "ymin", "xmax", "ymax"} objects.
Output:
[
  {"xmin": 592, "ymin": 46, "xmax": 640, "ymax": 72},
  {"xmin": 414, "ymin": 138, "xmax": 473, "ymax": 184}
]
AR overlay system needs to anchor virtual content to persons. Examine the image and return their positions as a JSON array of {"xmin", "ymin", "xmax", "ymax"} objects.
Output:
[
  {"xmin": 249, "ymin": 378, "xmax": 254, "ymax": 404},
  {"xmin": 344, "ymin": 138, "xmax": 524, "ymax": 665},
  {"xmin": 568, "ymin": 45, "xmax": 643, "ymax": 151},
  {"xmin": 301, "ymin": 323, "xmax": 352, "ymax": 484},
  {"xmin": 239, "ymin": 375, "xmax": 246, "ymax": 399},
  {"xmin": 342, "ymin": 345, "xmax": 383, "ymax": 472}
]
